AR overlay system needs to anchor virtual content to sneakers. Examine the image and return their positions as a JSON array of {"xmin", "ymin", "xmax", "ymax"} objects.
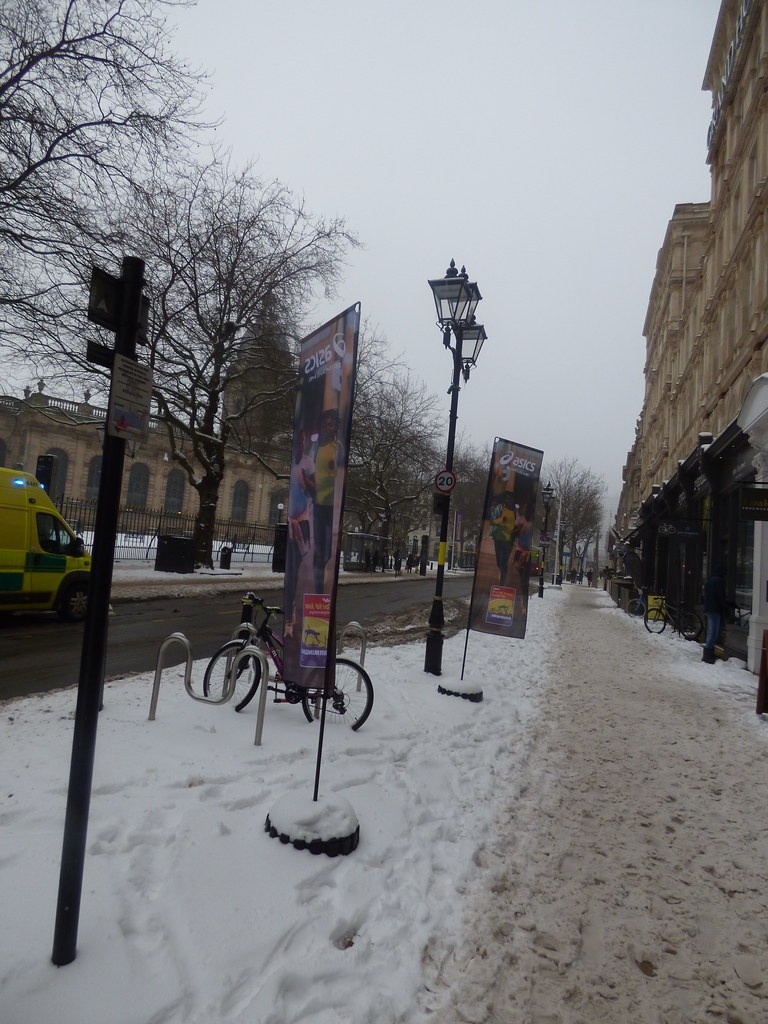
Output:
[
  {"xmin": 289, "ymin": 602, "xmax": 296, "ymax": 624},
  {"xmin": 284, "ymin": 622, "xmax": 294, "ymax": 639},
  {"xmin": 521, "ymin": 607, "xmax": 526, "ymax": 615},
  {"xmin": 520, "ymin": 599, "xmax": 524, "ymax": 609}
]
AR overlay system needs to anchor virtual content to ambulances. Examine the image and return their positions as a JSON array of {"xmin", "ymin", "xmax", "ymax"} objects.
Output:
[{"xmin": 0, "ymin": 464, "xmax": 96, "ymax": 623}]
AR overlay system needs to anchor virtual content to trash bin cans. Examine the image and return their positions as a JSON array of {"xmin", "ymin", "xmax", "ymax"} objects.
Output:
[{"xmin": 154, "ymin": 534, "xmax": 196, "ymax": 575}]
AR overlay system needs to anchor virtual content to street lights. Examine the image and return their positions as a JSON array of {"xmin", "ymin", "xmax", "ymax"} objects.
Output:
[
  {"xmin": 422, "ymin": 256, "xmax": 489, "ymax": 676},
  {"xmin": 277, "ymin": 503, "xmax": 285, "ymax": 522},
  {"xmin": 537, "ymin": 480, "xmax": 555, "ymax": 598}
]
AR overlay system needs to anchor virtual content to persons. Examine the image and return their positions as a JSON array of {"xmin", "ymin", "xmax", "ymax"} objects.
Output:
[
  {"xmin": 361, "ymin": 544, "xmax": 422, "ymax": 579},
  {"xmin": 568, "ymin": 566, "xmax": 614, "ymax": 588},
  {"xmin": 487, "ymin": 486, "xmax": 533, "ymax": 617},
  {"xmin": 283, "ymin": 407, "xmax": 347, "ymax": 653},
  {"xmin": 230, "ymin": 533, "xmax": 238, "ymax": 553},
  {"xmin": 701, "ymin": 563, "xmax": 729, "ymax": 666}
]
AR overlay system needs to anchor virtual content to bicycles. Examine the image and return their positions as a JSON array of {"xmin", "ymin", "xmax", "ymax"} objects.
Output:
[
  {"xmin": 626, "ymin": 584, "xmax": 671, "ymax": 621},
  {"xmin": 644, "ymin": 595, "xmax": 703, "ymax": 641},
  {"xmin": 202, "ymin": 591, "xmax": 376, "ymax": 733}
]
